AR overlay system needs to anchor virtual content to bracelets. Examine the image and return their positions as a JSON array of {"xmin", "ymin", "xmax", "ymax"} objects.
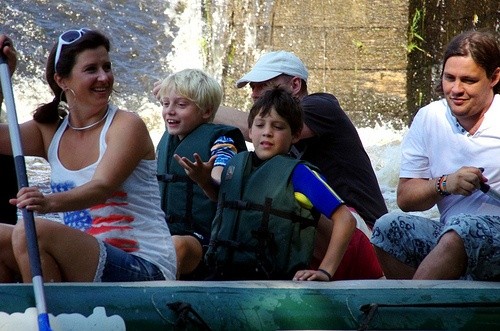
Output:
[
  {"xmin": 317, "ymin": 269, "xmax": 332, "ymax": 280},
  {"xmin": 435, "ymin": 175, "xmax": 450, "ymax": 197}
]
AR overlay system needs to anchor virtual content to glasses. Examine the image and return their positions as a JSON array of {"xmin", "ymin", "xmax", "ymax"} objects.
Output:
[{"xmin": 55, "ymin": 28, "xmax": 92, "ymax": 73}]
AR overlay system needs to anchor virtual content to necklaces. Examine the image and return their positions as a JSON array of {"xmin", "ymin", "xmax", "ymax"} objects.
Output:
[
  {"xmin": 451, "ymin": 112, "xmax": 472, "ymax": 136},
  {"xmin": 68, "ymin": 108, "xmax": 109, "ymax": 130}
]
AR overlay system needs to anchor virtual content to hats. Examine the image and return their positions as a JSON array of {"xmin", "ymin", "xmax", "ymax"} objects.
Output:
[{"xmin": 237, "ymin": 51, "xmax": 308, "ymax": 89}]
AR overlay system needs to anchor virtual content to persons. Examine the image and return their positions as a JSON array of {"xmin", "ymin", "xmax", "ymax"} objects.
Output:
[
  {"xmin": 155, "ymin": 68, "xmax": 248, "ymax": 281},
  {"xmin": 370, "ymin": 31, "xmax": 500, "ymax": 281},
  {"xmin": 153, "ymin": 50, "xmax": 389, "ymax": 280},
  {"xmin": 0, "ymin": 28, "xmax": 176, "ymax": 283},
  {"xmin": 201, "ymin": 87, "xmax": 356, "ymax": 281}
]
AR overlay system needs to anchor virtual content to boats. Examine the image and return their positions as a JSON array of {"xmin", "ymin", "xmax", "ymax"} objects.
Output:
[{"xmin": 0, "ymin": 277, "xmax": 500, "ymax": 331}]
